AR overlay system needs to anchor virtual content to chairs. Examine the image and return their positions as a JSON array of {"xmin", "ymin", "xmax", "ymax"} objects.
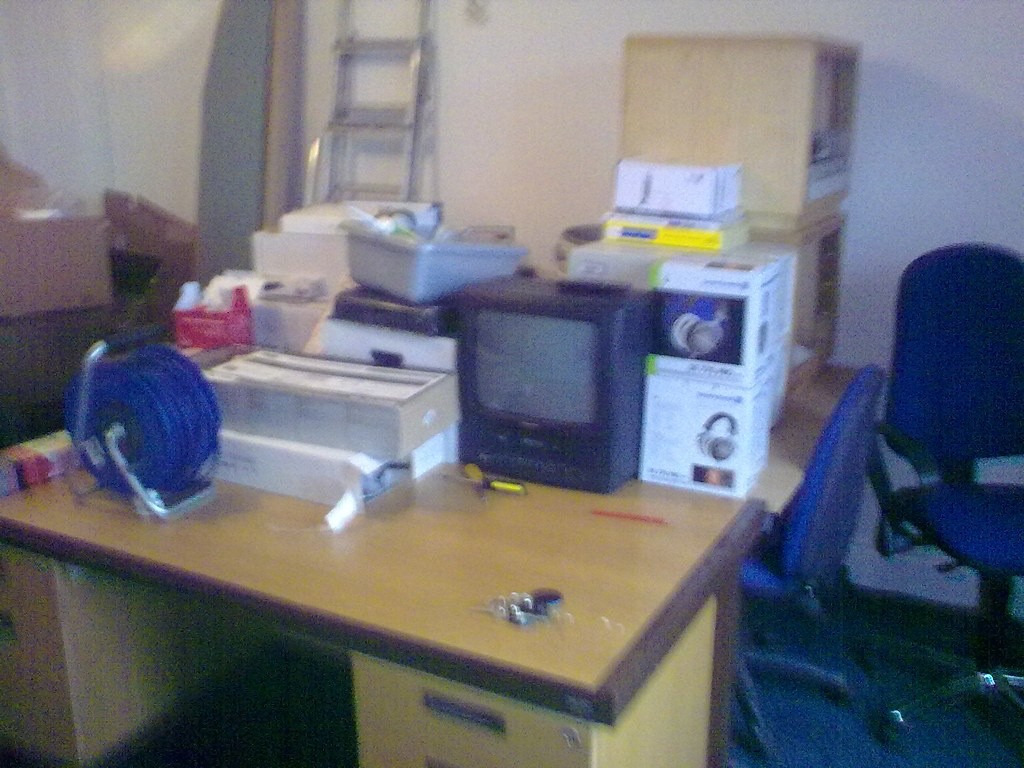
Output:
[
  {"xmin": 867, "ymin": 242, "xmax": 1024, "ymax": 744},
  {"xmin": 728, "ymin": 363, "xmax": 886, "ymax": 768}
]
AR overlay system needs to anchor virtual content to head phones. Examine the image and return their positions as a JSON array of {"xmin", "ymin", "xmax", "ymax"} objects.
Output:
[
  {"xmin": 668, "ymin": 297, "xmax": 729, "ymax": 356},
  {"xmin": 698, "ymin": 412, "xmax": 739, "ymax": 461}
]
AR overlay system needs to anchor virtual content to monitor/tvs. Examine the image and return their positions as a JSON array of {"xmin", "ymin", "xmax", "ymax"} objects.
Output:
[{"xmin": 453, "ymin": 274, "xmax": 652, "ymax": 492}]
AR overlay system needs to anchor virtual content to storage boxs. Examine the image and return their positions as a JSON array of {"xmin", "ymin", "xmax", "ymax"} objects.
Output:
[
  {"xmin": 750, "ymin": 213, "xmax": 845, "ymax": 399},
  {"xmin": 194, "ymin": 345, "xmax": 460, "ymax": 462},
  {"xmin": 337, "ymin": 218, "xmax": 530, "ymax": 307},
  {"xmin": 611, "ymin": 152, "xmax": 744, "ymax": 218},
  {"xmin": 0, "ymin": 157, "xmax": 112, "ymax": 318},
  {"xmin": 0, "ymin": 430, "xmax": 80, "ymax": 497},
  {"xmin": 199, "ymin": 429, "xmax": 446, "ymax": 531},
  {"xmin": 618, "ymin": 34, "xmax": 861, "ymax": 233},
  {"xmin": 637, "ymin": 256, "xmax": 782, "ymax": 498},
  {"xmin": 745, "ymin": 239, "xmax": 797, "ymax": 432},
  {"xmin": 278, "ymin": 200, "xmax": 441, "ymax": 237}
]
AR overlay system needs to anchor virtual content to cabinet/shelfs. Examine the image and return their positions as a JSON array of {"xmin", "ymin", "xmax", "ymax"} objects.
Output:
[{"xmin": 0, "ymin": 431, "xmax": 767, "ymax": 768}]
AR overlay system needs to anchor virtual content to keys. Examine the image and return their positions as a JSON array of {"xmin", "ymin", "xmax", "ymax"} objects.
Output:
[{"xmin": 471, "ymin": 590, "xmax": 534, "ymax": 626}]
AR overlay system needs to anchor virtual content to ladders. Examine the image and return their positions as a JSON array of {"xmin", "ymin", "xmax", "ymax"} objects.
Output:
[{"xmin": 324, "ymin": 0, "xmax": 436, "ymax": 204}]
